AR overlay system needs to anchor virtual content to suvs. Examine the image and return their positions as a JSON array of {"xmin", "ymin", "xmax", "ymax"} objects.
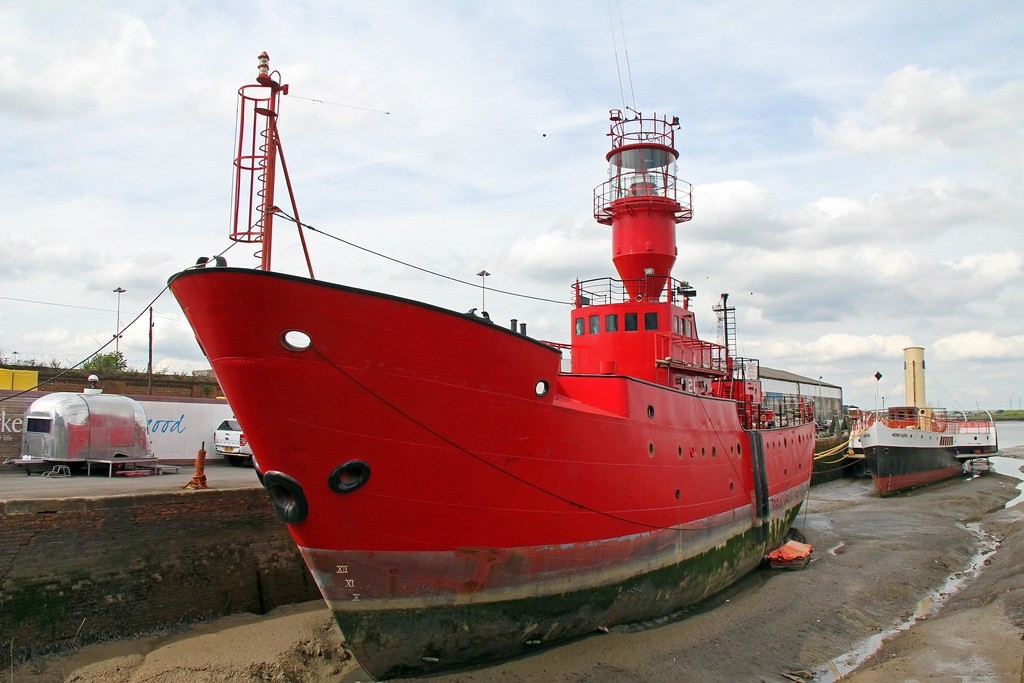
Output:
[{"xmin": 213, "ymin": 415, "xmax": 254, "ymax": 465}]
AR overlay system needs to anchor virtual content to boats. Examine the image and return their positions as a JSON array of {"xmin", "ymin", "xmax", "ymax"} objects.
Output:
[
  {"xmin": 167, "ymin": 50, "xmax": 820, "ymax": 682},
  {"xmin": 843, "ymin": 360, "xmax": 1003, "ymax": 498}
]
